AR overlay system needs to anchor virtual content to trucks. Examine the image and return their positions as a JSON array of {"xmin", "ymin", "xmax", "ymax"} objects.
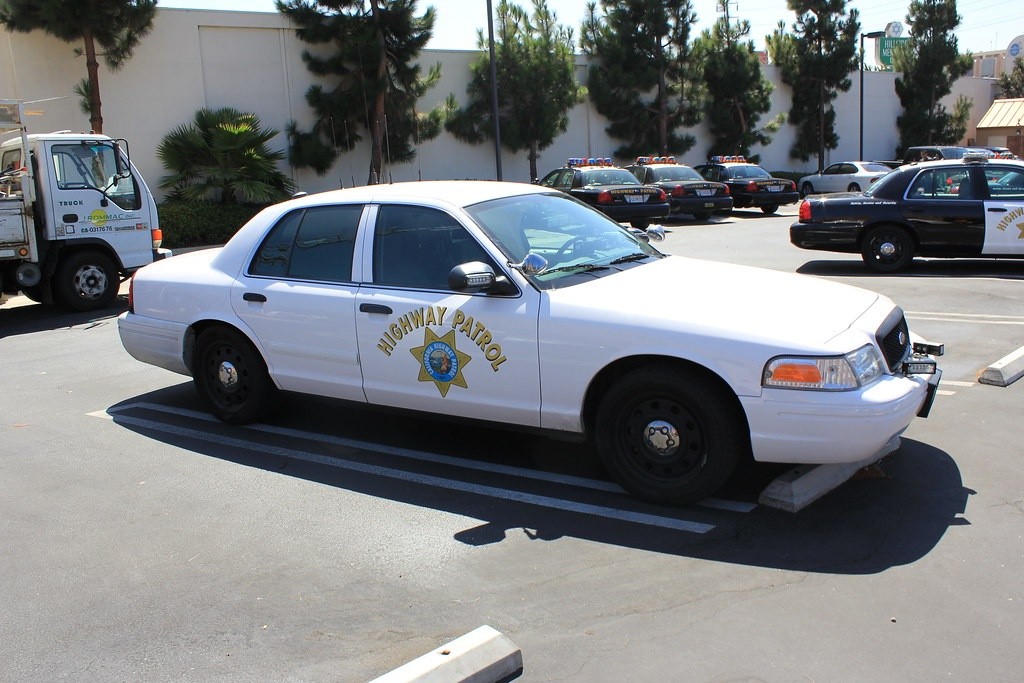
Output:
[{"xmin": 0, "ymin": 96, "xmax": 173, "ymax": 312}]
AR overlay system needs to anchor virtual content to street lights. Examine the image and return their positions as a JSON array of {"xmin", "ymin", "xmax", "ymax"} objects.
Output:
[{"xmin": 858, "ymin": 30, "xmax": 887, "ymax": 162}]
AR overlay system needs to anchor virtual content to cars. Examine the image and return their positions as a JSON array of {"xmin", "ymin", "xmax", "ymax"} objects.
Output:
[
  {"xmin": 692, "ymin": 155, "xmax": 800, "ymax": 214},
  {"xmin": 117, "ymin": 180, "xmax": 946, "ymax": 507},
  {"xmin": 797, "ymin": 160, "xmax": 893, "ymax": 194},
  {"xmin": 529, "ymin": 158, "xmax": 671, "ymax": 231},
  {"xmin": 621, "ymin": 156, "xmax": 735, "ymax": 221},
  {"xmin": 788, "ymin": 151, "xmax": 1024, "ymax": 273},
  {"xmin": 868, "ymin": 145, "xmax": 1023, "ymax": 167}
]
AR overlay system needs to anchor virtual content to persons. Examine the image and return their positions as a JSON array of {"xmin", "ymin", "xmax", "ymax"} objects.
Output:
[{"xmin": 919, "ymin": 150, "xmax": 932, "ymax": 161}]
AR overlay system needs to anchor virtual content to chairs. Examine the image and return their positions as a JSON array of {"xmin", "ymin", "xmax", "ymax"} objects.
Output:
[
  {"xmin": 397, "ymin": 222, "xmax": 451, "ymax": 288},
  {"xmin": 453, "ymin": 226, "xmax": 487, "ymax": 264},
  {"xmin": 957, "ymin": 178, "xmax": 972, "ymax": 199}
]
